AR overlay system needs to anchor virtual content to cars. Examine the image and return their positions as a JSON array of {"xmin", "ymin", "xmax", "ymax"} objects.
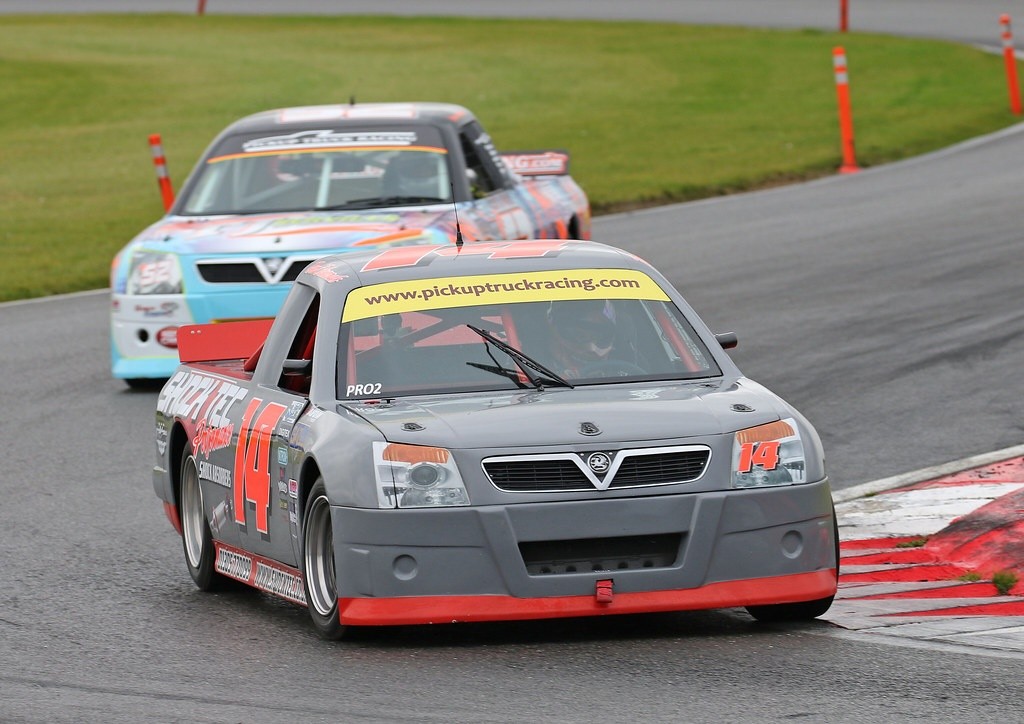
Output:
[
  {"xmin": 108, "ymin": 101, "xmax": 589, "ymax": 392},
  {"xmin": 150, "ymin": 238, "xmax": 842, "ymax": 642}
]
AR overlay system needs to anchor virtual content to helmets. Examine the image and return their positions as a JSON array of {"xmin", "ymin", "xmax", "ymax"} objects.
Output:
[
  {"xmin": 393, "ymin": 148, "xmax": 447, "ymax": 194},
  {"xmin": 544, "ymin": 297, "xmax": 618, "ymax": 370}
]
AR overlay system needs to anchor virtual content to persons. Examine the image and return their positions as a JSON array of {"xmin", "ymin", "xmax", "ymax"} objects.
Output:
[
  {"xmin": 525, "ymin": 294, "xmax": 645, "ymax": 385},
  {"xmin": 395, "ymin": 148, "xmax": 439, "ymax": 198}
]
